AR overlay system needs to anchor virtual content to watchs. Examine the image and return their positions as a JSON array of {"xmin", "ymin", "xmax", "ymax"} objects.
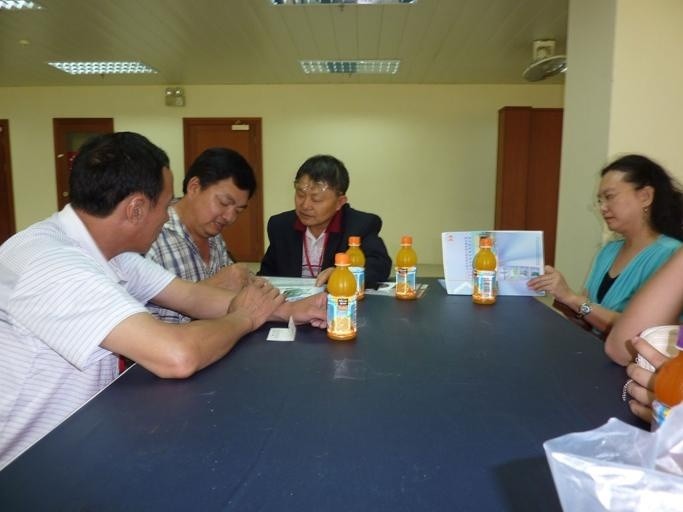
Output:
[{"xmin": 576, "ymin": 301, "xmax": 593, "ymax": 319}]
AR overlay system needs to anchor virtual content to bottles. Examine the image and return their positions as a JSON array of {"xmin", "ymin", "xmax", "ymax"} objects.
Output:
[
  {"xmin": 325, "ymin": 253, "xmax": 358, "ymax": 341},
  {"xmin": 472, "ymin": 235, "xmax": 497, "ymax": 305},
  {"xmin": 648, "ymin": 327, "xmax": 683, "ymax": 432},
  {"xmin": 343, "ymin": 237, "xmax": 366, "ymax": 301},
  {"xmin": 393, "ymin": 237, "xmax": 419, "ymax": 300}
]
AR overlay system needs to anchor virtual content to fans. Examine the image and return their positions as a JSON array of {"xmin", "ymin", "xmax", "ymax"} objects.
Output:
[{"xmin": 522, "ymin": 41, "xmax": 567, "ymax": 84}]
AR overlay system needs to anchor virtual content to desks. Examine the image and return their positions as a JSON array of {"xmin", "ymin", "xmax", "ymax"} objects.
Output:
[{"xmin": 0, "ymin": 277, "xmax": 654, "ymax": 511}]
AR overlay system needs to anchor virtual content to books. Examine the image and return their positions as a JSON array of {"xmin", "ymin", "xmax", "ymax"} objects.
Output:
[
  {"xmin": 257, "ymin": 274, "xmax": 329, "ymax": 301},
  {"xmin": 436, "ymin": 230, "xmax": 546, "ymax": 297}
]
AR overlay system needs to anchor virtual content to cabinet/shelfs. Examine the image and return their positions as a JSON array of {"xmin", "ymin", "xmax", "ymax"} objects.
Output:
[{"xmin": 492, "ymin": 104, "xmax": 564, "ymax": 272}]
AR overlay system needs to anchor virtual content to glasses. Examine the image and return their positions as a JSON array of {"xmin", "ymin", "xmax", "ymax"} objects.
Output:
[
  {"xmin": 592, "ymin": 186, "xmax": 645, "ymax": 208},
  {"xmin": 293, "ymin": 179, "xmax": 343, "ymax": 195}
]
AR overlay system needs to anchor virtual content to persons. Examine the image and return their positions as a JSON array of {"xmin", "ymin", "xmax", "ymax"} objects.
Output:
[
  {"xmin": 256, "ymin": 155, "xmax": 392, "ymax": 282},
  {"xmin": 0, "ymin": 132, "xmax": 327, "ymax": 471},
  {"xmin": 136, "ymin": 147, "xmax": 256, "ymax": 324},
  {"xmin": 526, "ymin": 154, "xmax": 682, "ymax": 338},
  {"xmin": 625, "ymin": 333, "xmax": 672, "ymax": 424}
]
